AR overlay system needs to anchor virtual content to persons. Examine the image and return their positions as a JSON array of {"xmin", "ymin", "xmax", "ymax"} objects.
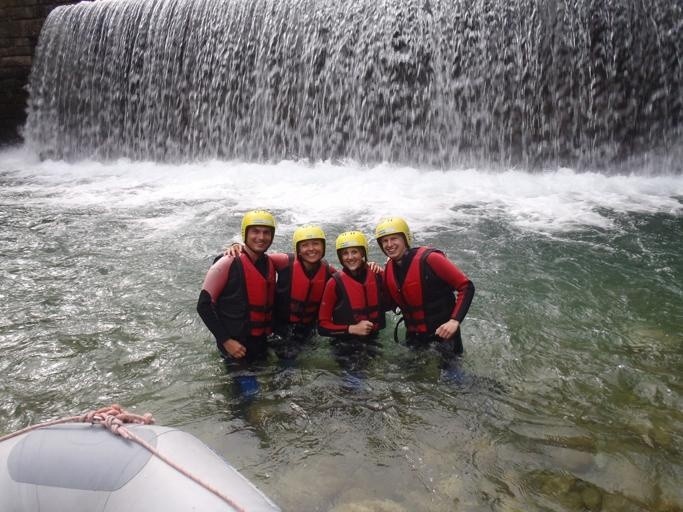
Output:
[
  {"xmin": 374, "ymin": 217, "xmax": 475, "ymax": 379},
  {"xmin": 317, "ymin": 230, "xmax": 395, "ymax": 385},
  {"xmin": 196, "ymin": 210, "xmax": 276, "ymax": 409},
  {"xmin": 222, "ymin": 223, "xmax": 383, "ymax": 399}
]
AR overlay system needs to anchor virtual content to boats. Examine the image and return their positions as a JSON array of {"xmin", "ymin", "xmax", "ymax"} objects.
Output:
[{"xmin": 1, "ymin": 414, "xmax": 282, "ymax": 512}]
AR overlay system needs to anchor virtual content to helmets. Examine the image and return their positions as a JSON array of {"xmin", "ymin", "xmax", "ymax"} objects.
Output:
[
  {"xmin": 241, "ymin": 210, "xmax": 276, "ymax": 252},
  {"xmin": 335, "ymin": 231, "xmax": 369, "ymax": 267},
  {"xmin": 293, "ymin": 225, "xmax": 326, "ymax": 258},
  {"xmin": 374, "ymin": 216, "xmax": 412, "ymax": 257}
]
